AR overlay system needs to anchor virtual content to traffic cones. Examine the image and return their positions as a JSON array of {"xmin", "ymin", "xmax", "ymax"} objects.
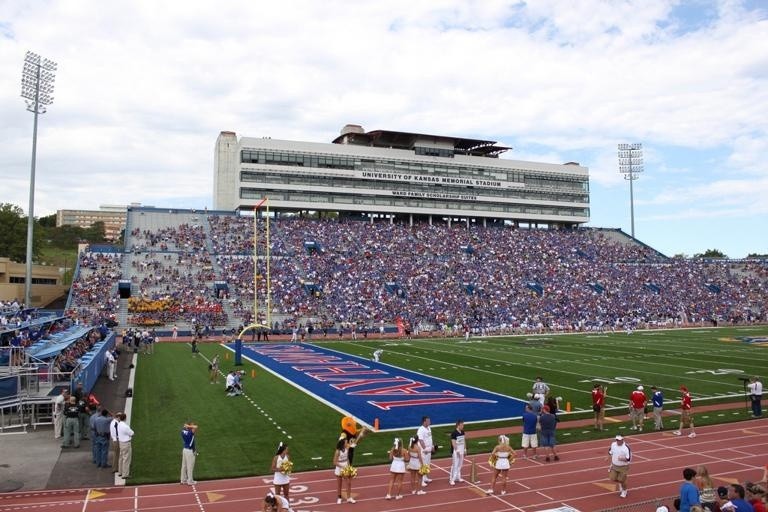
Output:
[{"xmin": 469, "ymin": 456, "xmax": 481, "ymax": 484}]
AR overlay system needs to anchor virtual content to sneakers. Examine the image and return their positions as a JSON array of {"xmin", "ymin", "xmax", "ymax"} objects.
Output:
[
  {"xmin": 687, "ymin": 432, "xmax": 696, "ymax": 438},
  {"xmin": 347, "ymin": 497, "xmax": 356, "ymax": 504},
  {"xmin": 501, "ymin": 489, "xmax": 507, "ymax": 494},
  {"xmin": 449, "ymin": 480, "xmax": 456, "ymax": 486},
  {"xmin": 458, "ymin": 479, "xmax": 464, "ymax": 483},
  {"xmin": 485, "ymin": 488, "xmax": 494, "ymax": 494},
  {"xmin": 619, "ymin": 482, "xmax": 627, "ymax": 497},
  {"xmin": 412, "ymin": 477, "xmax": 432, "ymax": 495},
  {"xmin": 336, "ymin": 498, "xmax": 342, "ymax": 504},
  {"xmin": 629, "ymin": 425, "xmax": 637, "ymax": 430},
  {"xmin": 395, "ymin": 494, "xmax": 403, "ymax": 500},
  {"xmin": 384, "ymin": 493, "xmax": 391, "ymax": 500},
  {"xmin": 522, "ymin": 454, "xmax": 558, "ymax": 462},
  {"xmin": 673, "ymin": 431, "xmax": 682, "ymax": 436}
]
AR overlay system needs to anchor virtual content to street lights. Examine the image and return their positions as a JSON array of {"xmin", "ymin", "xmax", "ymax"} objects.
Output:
[
  {"xmin": 19, "ymin": 47, "xmax": 59, "ymax": 308},
  {"xmin": 617, "ymin": 143, "xmax": 646, "ymax": 239}
]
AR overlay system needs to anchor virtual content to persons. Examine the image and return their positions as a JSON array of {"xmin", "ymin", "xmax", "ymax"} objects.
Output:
[
  {"xmin": 748, "ymin": 376, "xmax": 764, "ymax": 420},
  {"xmin": 520, "ymin": 376, "xmax": 699, "ymax": 498},
  {"xmin": 263, "ymin": 413, "xmax": 515, "ymax": 512},
  {"xmin": 65, "ymin": 217, "xmax": 767, "ymax": 396},
  {"xmin": 654, "ymin": 467, "xmax": 767, "ymax": 511},
  {"xmin": 5, "ymin": 298, "xmax": 134, "ymax": 481},
  {"xmin": 179, "ymin": 420, "xmax": 199, "ymax": 487}
]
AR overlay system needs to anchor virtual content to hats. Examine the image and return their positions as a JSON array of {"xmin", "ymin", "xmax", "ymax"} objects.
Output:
[
  {"xmin": 637, "ymin": 385, "xmax": 643, "ymax": 390},
  {"xmin": 615, "ymin": 435, "xmax": 624, "ymax": 440}
]
